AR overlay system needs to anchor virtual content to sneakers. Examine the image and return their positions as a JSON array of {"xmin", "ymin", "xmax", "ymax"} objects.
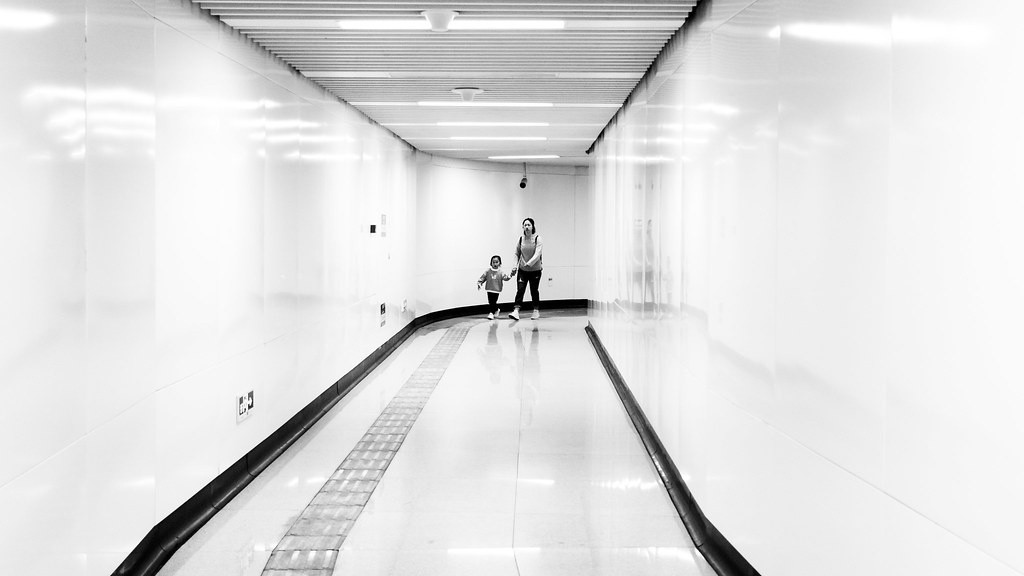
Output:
[
  {"xmin": 531, "ymin": 310, "xmax": 540, "ymax": 320},
  {"xmin": 509, "ymin": 310, "xmax": 519, "ymax": 320}
]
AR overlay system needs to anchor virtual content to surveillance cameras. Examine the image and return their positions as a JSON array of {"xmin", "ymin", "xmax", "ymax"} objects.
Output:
[{"xmin": 520, "ymin": 177, "xmax": 527, "ymax": 189}]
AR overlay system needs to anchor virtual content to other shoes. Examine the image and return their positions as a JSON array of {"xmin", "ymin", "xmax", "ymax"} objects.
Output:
[
  {"xmin": 487, "ymin": 313, "xmax": 494, "ymax": 320},
  {"xmin": 494, "ymin": 309, "xmax": 500, "ymax": 317}
]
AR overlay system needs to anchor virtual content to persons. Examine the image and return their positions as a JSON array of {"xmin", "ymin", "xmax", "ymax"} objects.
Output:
[
  {"xmin": 477, "ymin": 255, "xmax": 515, "ymax": 319},
  {"xmin": 507, "ymin": 218, "xmax": 543, "ymax": 320}
]
